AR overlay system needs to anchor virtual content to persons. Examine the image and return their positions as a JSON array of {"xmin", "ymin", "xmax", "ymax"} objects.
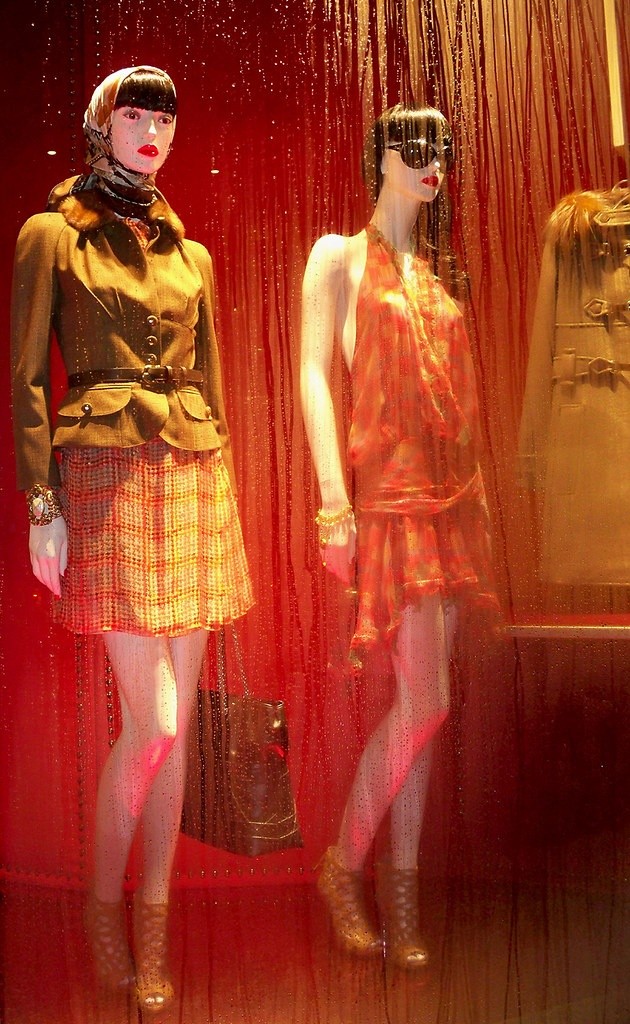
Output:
[
  {"xmin": 11, "ymin": 64, "xmax": 254, "ymax": 1009},
  {"xmin": 299, "ymin": 101, "xmax": 504, "ymax": 968}
]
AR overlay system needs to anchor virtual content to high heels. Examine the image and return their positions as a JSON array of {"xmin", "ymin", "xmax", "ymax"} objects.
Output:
[
  {"xmin": 127, "ymin": 892, "xmax": 174, "ymax": 1016},
  {"xmin": 372, "ymin": 858, "xmax": 433, "ymax": 972},
  {"xmin": 84, "ymin": 888, "xmax": 134, "ymax": 997},
  {"xmin": 313, "ymin": 846, "xmax": 384, "ymax": 960}
]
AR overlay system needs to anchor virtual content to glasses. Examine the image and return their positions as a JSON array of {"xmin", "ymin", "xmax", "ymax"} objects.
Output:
[{"xmin": 384, "ymin": 137, "xmax": 456, "ymax": 175}]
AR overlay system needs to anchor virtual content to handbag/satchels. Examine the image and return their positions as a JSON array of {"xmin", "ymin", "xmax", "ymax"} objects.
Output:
[{"xmin": 179, "ymin": 621, "xmax": 304, "ymax": 856}]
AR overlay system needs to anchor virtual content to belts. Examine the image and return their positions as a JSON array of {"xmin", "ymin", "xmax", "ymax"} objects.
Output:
[{"xmin": 69, "ymin": 364, "xmax": 204, "ymax": 394}]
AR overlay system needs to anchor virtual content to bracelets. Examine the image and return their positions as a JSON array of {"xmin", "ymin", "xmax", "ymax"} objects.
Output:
[{"xmin": 315, "ymin": 506, "xmax": 352, "ymax": 560}]
